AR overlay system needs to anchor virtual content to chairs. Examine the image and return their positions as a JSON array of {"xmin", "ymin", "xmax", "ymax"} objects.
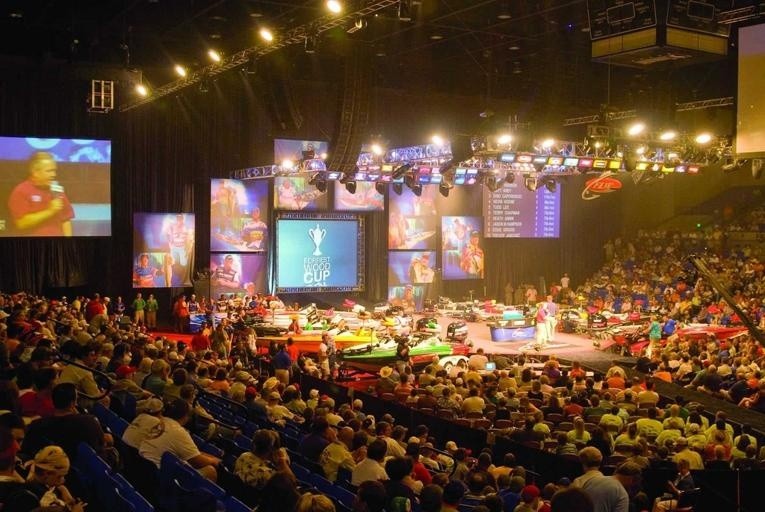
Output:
[{"xmin": 0, "ymin": 233, "xmax": 765, "ymax": 510}]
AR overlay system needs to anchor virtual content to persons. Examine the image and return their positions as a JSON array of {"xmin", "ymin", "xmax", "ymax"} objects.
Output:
[
  {"xmin": 212, "ymin": 178, "xmax": 230, "ymax": 230},
  {"xmin": 278, "ymin": 180, "xmax": 297, "ymax": 206},
  {"xmin": 5, "ymin": 150, "xmax": 75, "ymax": 235},
  {"xmin": 0, "ymin": 202, "xmax": 765, "ymax": 512}
]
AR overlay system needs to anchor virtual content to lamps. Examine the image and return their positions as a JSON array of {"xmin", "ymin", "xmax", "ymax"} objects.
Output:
[{"xmin": 310, "ymin": 140, "xmax": 764, "ymax": 198}]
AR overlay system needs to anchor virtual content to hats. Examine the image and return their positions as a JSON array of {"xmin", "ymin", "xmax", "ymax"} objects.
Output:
[
  {"xmin": 392, "ymin": 425, "xmax": 409, "ymax": 436},
  {"xmin": 576, "ymin": 446, "xmax": 604, "ymax": 465},
  {"xmin": 328, "ymin": 413, "xmax": 346, "ymax": 429},
  {"xmin": 309, "ymin": 388, "xmax": 320, "ymax": 398},
  {"xmin": 244, "ymin": 386, "xmax": 257, "ymax": 400},
  {"xmin": 407, "ymin": 436, "xmax": 421, "ymax": 444},
  {"xmin": 379, "ymin": 365, "xmax": 394, "ymax": 378},
  {"xmin": 520, "ymin": 484, "xmax": 541, "ymax": 504},
  {"xmin": 169, "ymin": 351, "xmax": 179, "ymax": 361},
  {"xmin": 445, "ymin": 440, "xmax": 459, "ymax": 451},
  {"xmin": 268, "ymin": 391, "xmax": 283, "ymax": 402},
  {"xmin": 103, "ymin": 297, "xmax": 112, "ymax": 303}
]
